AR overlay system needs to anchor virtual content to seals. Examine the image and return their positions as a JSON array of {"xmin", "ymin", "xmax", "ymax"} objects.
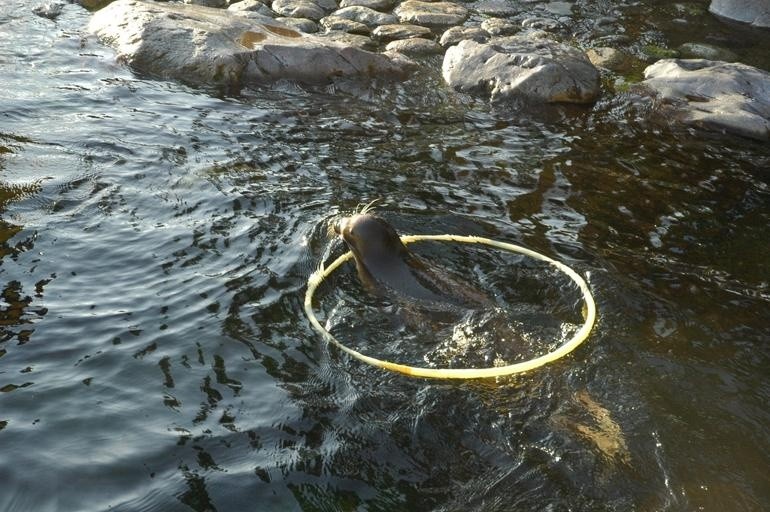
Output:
[{"xmin": 325, "ymin": 198, "xmax": 630, "ymax": 464}]
